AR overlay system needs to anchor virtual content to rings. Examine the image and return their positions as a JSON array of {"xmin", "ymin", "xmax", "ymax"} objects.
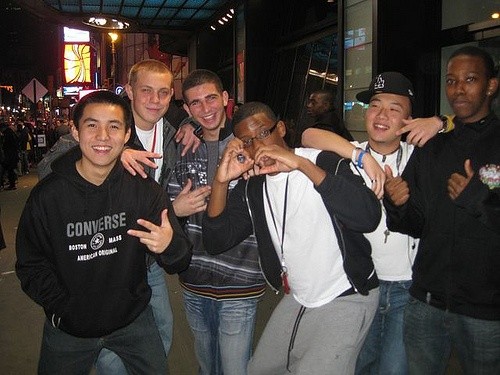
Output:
[{"xmin": 372, "ymin": 180, "xmax": 377, "ymax": 182}]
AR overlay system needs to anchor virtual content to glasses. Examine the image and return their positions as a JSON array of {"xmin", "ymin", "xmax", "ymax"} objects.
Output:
[{"xmin": 244, "ymin": 123, "xmax": 277, "ymax": 148}]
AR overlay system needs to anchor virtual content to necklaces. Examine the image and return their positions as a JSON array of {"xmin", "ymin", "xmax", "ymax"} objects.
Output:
[
  {"xmin": 265, "ymin": 175, "xmax": 290, "ymax": 295},
  {"xmin": 366, "ymin": 143, "xmax": 403, "ymax": 243},
  {"xmin": 151, "ymin": 124, "xmax": 157, "ymax": 154}
]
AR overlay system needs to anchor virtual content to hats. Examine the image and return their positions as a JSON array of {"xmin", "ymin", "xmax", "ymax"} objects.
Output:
[{"xmin": 355, "ymin": 72, "xmax": 415, "ymax": 105}]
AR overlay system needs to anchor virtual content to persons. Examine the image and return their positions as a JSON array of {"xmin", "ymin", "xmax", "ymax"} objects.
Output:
[
  {"xmin": 203, "ymin": 102, "xmax": 382, "ymax": 375},
  {"xmin": 0, "ymin": 116, "xmax": 74, "ymax": 191},
  {"xmin": 38, "ymin": 58, "xmax": 200, "ymax": 375},
  {"xmin": 14, "ymin": 91, "xmax": 194, "ymax": 375},
  {"xmin": 301, "ymin": 73, "xmax": 455, "ymax": 375},
  {"xmin": 307, "ymin": 90, "xmax": 353, "ymax": 141},
  {"xmin": 167, "ymin": 69, "xmax": 265, "ymax": 375},
  {"xmin": 383, "ymin": 45, "xmax": 500, "ymax": 375}
]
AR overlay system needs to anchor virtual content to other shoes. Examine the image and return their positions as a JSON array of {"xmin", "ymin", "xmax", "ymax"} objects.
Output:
[{"xmin": 4, "ymin": 186, "xmax": 16, "ymax": 190}]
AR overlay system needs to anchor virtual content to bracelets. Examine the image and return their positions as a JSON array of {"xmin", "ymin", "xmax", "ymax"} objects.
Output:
[
  {"xmin": 358, "ymin": 150, "xmax": 368, "ymax": 168},
  {"xmin": 352, "ymin": 147, "xmax": 362, "ymax": 165},
  {"xmin": 436, "ymin": 114, "xmax": 453, "ymax": 133}
]
